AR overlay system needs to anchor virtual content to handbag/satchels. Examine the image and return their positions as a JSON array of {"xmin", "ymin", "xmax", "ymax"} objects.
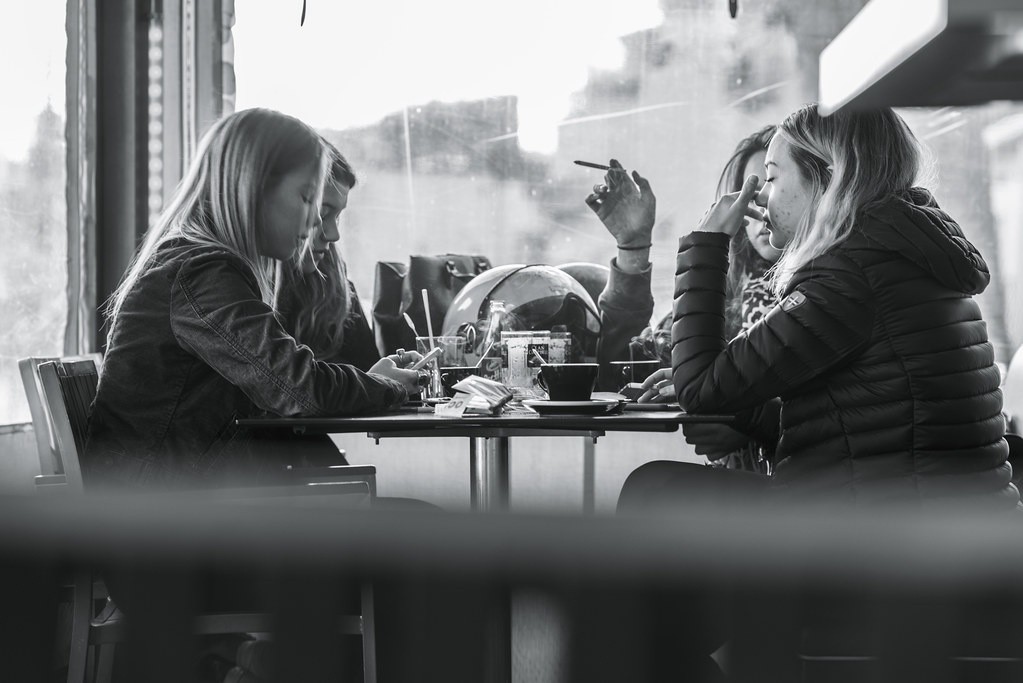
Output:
[{"xmin": 371, "ymin": 255, "xmax": 490, "ymax": 357}]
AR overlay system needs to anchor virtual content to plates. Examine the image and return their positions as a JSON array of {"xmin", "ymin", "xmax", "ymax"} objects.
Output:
[{"xmin": 521, "ymin": 398, "xmax": 620, "ymax": 416}]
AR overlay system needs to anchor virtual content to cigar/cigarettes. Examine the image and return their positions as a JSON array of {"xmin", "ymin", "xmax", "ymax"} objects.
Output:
[{"xmin": 574, "ymin": 160, "xmax": 627, "ymax": 174}]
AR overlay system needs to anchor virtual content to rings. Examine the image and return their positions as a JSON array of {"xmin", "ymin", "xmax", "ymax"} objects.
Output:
[
  {"xmin": 417, "ymin": 372, "xmax": 428, "ymax": 393},
  {"xmin": 396, "ymin": 348, "xmax": 405, "ymax": 362}
]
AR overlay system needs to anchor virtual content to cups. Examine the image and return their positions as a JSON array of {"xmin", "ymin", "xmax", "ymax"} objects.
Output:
[
  {"xmin": 610, "ymin": 360, "xmax": 661, "ymax": 391},
  {"xmin": 416, "ymin": 335, "xmax": 469, "ymax": 406},
  {"xmin": 440, "ymin": 366, "xmax": 480, "ymax": 398},
  {"xmin": 537, "ymin": 362, "xmax": 599, "ymax": 401}
]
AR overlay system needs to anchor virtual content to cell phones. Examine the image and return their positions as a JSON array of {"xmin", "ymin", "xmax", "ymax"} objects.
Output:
[
  {"xmin": 410, "ymin": 346, "xmax": 441, "ymax": 370},
  {"xmin": 622, "ymin": 402, "xmax": 680, "ymax": 411}
]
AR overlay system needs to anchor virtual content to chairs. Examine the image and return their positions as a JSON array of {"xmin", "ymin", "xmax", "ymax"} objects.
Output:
[
  {"xmin": 17, "ymin": 355, "xmax": 382, "ymax": 683},
  {"xmin": 36, "ymin": 358, "xmax": 379, "ymax": 683}
]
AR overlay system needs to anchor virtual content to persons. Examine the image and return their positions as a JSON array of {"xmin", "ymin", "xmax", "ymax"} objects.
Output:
[
  {"xmin": 585, "ymin": 102, "xmax": 1019, "ymax": 682},
  {"xmin": 85, "ymin": 108, "xmax": 431, "ymax": 683}
]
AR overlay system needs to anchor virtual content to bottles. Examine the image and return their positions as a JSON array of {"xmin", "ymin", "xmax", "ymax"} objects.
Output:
[{"xmin": 481, "ymin": 300, "xmax": 512, "ymax": 385}]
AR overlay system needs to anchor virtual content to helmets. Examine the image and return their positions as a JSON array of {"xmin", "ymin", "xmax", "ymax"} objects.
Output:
[
  {"xmin": 442, "ymin": 264, "xmax": 603, "ymax": 368},
  {"xmin": 556, "ymin": 258, "xmax": 611, "ymax": 304}
]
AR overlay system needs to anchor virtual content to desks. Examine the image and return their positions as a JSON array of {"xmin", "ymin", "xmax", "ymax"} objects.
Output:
[{"xmin": 231, "ymin": 389, "xmax": 730, "ymax": 683}]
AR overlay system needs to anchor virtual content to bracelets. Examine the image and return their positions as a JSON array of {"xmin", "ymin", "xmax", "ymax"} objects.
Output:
[{"xmin": 617, "ymin": 244, "xmax": 653, "ymax": 251}]
